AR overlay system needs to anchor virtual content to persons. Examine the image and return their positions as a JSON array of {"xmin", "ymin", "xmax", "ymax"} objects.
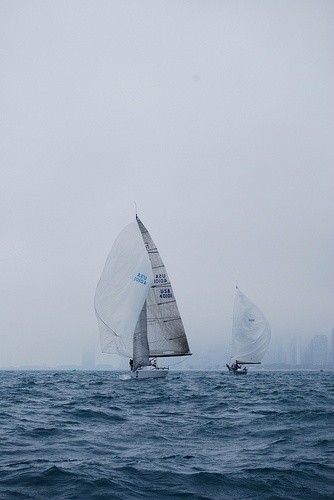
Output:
[{"xmin": 130, "ymin": 359, "xmax": 133, "ymax": 370}]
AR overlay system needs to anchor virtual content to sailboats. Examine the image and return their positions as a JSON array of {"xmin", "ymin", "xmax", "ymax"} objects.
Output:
[
  {"xmin": 92, "ymin": 211, "xmax": 193, "ymax": 380},
  {"xmin": 226, "ymin": 285, "xmax": 272, "ymax": 376}
]
